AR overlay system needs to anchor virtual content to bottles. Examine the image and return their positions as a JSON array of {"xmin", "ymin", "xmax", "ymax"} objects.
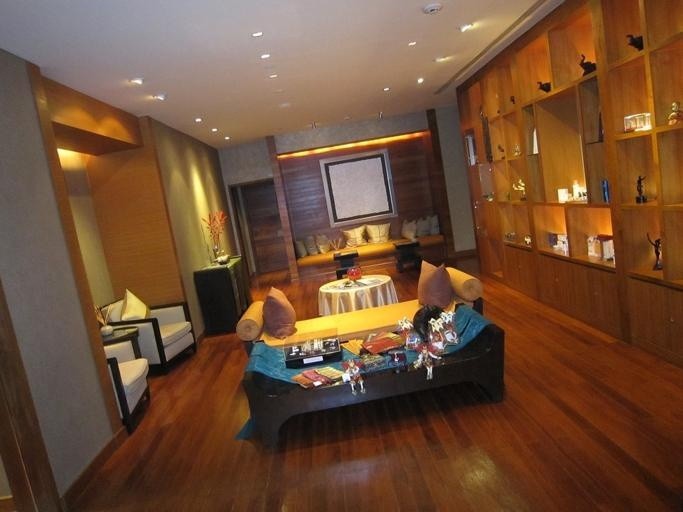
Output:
[
  {"xmin": 624, "ymin": 113, "xmax": 651, "ymax": 131},
  {"xmin": 603, "ymin": 179, "xmax": 609, "ymax": 201},
  {"xmin": 572, "ymin": 180, "xmax": 588, "ymax": 202},
  {"xmin": 532, "ymin": 126, "xmax": 539, "ymax": 154}
]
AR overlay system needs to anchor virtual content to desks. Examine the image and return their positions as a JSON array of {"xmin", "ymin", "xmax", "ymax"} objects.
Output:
[{"xmin": 234, "ymin": 302, "xmax": 505, "ymax": 448}]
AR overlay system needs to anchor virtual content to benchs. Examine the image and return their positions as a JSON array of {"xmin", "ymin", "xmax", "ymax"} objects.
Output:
[{"xmin": 297, "ymin": 236, "xmax": 445, "ymax": 278}]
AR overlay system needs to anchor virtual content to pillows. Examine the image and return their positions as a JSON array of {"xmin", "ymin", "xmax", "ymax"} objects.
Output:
[
  {"xmin": 418, "ymin": 261, "xmax": 453, "ymax": 309},
  {"xmin": 263, "ymin": 287, "xmax": 296, "ymax": 340},
  {"xmin": 120, "ymin": 288, "xmax": 150, "ymax": 321},
  {"xmin": 294, "ymin": 215, "xmax": 439, "ymax": 260}
]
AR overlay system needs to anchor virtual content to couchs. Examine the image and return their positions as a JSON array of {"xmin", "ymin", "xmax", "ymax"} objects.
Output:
[{"xmin": 236, "ymin": 267, "xmax": 485, "ymax": 357}]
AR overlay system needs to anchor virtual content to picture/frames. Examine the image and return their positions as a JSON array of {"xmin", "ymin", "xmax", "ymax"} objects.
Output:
[{"xmin": 318, "ymin": 147, "xmax": 398, "ymax": 229}]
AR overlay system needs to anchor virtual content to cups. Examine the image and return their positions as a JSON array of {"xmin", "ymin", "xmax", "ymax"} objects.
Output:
[
  {"xmin": 549, "ymin": 234, "xmax": 557, "ymax": 246},
  {"xmin": 558, "ymin": 188, "xmax": 568, "ymax": 202}
]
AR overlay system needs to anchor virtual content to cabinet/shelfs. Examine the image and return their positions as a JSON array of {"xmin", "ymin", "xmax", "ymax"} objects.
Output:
[
  {"xmin": 193, "ymin": 255, "xmax": 252, "ymax": 335},
  {"xmin": 456, "ymin": 0, "xmax": 683, "ymax": 369}
]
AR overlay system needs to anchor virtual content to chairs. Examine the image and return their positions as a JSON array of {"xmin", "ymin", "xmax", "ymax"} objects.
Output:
[{"xmin": 98, "ymin": 296, "xmax": 196, "ymax": 434}]
